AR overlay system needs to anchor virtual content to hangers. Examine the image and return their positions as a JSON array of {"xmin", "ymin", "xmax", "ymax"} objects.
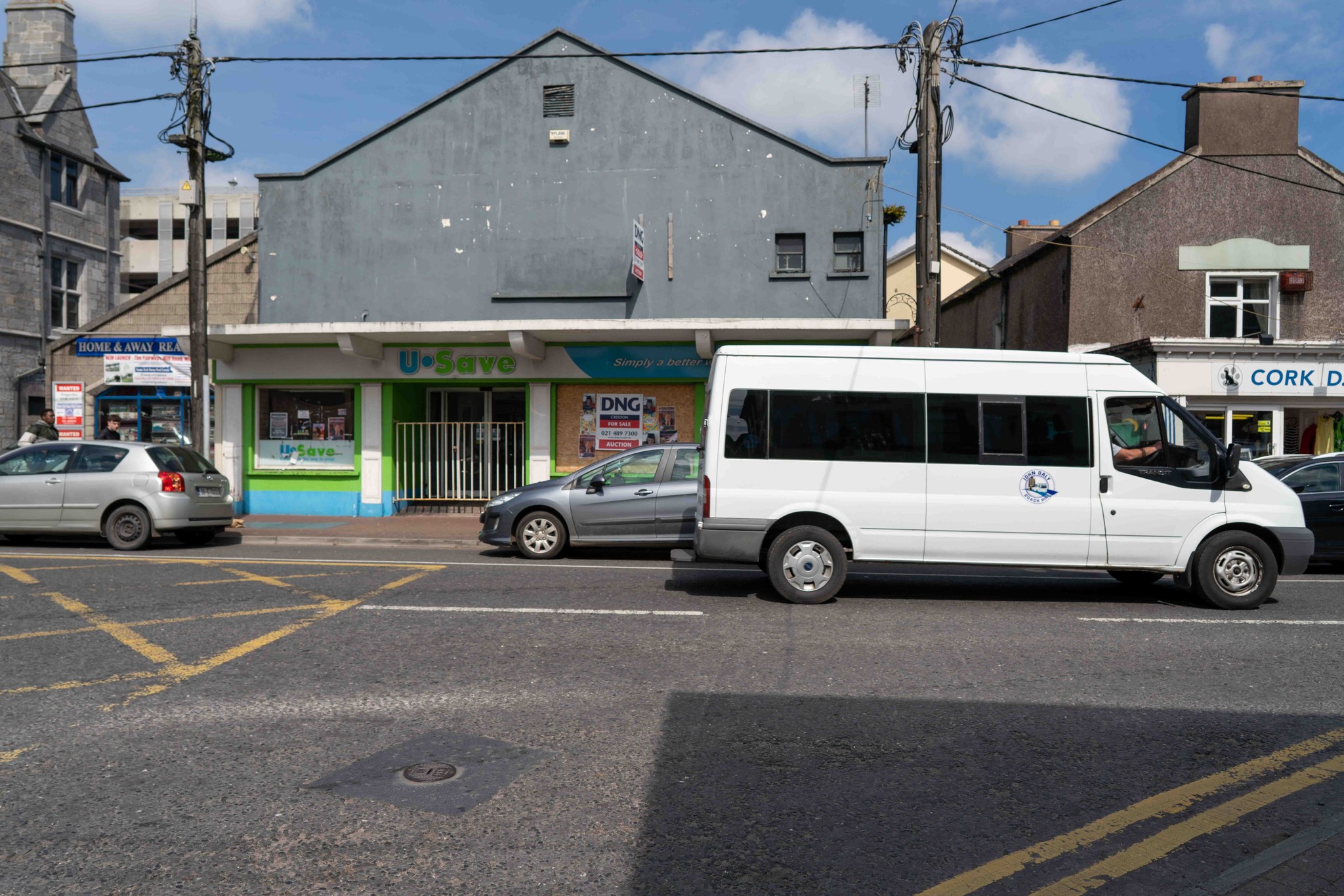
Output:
[
  {"xmin": 1310, "ymin": 411, "xmax": 1343, "ymax": 427},
  {"xmin": 1289, "ymin": 417, "xmax": 1296, "ymax": 426}
]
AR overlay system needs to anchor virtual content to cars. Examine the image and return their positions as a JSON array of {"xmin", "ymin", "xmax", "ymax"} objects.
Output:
[
  {"xmin": 1244, "ymin": 452, "xmax": 1344, "ymax": 571},
  {"xmin": 476, "ymin": 442, "xmax": 701, "ymax": 559},
  {"xmin": 0, "ymin": 440, "xmax": 234, "ymax": 552}
]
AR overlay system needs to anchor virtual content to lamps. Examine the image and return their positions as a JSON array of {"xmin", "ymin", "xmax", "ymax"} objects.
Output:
[
  {"xmin": 241, "ymin": 246, "xmax": 256, "ymax": 263},
  {"xmin": 1258, "ymin": 334, "xmax": 1273, "ymax": 345}
]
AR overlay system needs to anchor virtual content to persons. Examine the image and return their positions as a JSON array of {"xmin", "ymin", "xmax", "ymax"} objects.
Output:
[
  {"xmin": 1112, "ymin": 441, "xmax": 1162, "ymax": 463},
  {"xmin": 642, "ymin": 433, "xmax": 655, "ymax": 444},
  {"xmin": 579, "ymin": 440, "xmax": 594, "ymax": 458},
  {"xmin": 93, "ymin": 415, "xmax": 123, "ymax": 467},
  {"xmin": 664, "ymin": 415, "xmax": 674, "ymax": 425},
  {"xmin": 583, "ymin": 396, "xmax": 595, "ymax": 412},
  {"xmin": 643, "ymin": 399, "xmax": 656, "ymax": 414},
  {"xmin": 18, "ymin": 408, "xmax": 58, "ymax": 460}
]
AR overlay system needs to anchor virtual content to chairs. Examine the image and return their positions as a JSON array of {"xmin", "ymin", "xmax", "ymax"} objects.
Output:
[
  {"xmin": 67, "ymin": 457, "xmax": 87, "ymax": 470},
  {"xmin": 671, "ymin": 458, "xmax": 691, "ymax": 480}
]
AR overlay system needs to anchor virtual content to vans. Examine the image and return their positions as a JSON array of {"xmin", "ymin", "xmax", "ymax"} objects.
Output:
[{"xmin": 695, "ymin": 343, "xmax": 1314, "ymax": 609}]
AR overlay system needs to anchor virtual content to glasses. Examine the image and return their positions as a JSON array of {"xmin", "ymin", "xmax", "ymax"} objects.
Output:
[{"xmin": 48, "ymin": 414, "xmax": 55, "ymax": 417}]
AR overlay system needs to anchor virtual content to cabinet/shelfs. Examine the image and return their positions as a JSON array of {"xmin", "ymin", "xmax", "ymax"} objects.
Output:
[{"xmin": 100, "ymin": 400, "xmax": 191, "ymax": 446}]
